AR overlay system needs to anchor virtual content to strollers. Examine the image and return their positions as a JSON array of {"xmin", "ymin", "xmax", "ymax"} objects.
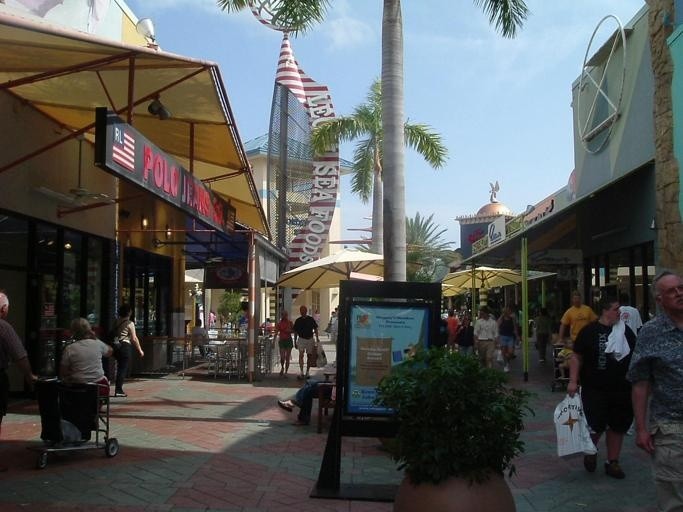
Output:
[{"xmin": 551, "ymin": 342, "xmax": 580, "ymax": 392}]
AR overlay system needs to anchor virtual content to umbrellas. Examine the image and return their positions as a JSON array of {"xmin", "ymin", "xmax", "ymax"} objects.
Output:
[
  {"xmin": 442, "ymin": 265, "xmax": 558, "ymax": 311},
  {"xmin": 272, "ymin": 245, "xmax": 384, "ymax": 291}
]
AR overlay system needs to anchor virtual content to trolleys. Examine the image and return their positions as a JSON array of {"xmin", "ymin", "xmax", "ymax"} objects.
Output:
[
  {"xmin": 207, "ymin": 336, "xmax": 276, "ymax": 379},
  {"xmin": 25, "ymin": 375, "xmax": 118, "ymax": 470}
]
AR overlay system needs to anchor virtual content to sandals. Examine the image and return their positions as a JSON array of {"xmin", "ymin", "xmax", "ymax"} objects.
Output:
[{"xmin": 278, "ymin": 400, "xmax": 308, "ymax": 426}]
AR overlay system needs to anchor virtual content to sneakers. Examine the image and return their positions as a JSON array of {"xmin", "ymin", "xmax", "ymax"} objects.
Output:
[
  {"xmin": 504, "ymin": 367, "xmax": 508, "ymax": 372},
  {"xmin": 280, "ymin": 367, "xmax": 309, "ymax": 379},
  {"xmin": 116, "ymin": 392, "xmax": 127, "ymax": 397},
  {"xmin": 605, "ymin": 460, "xmax": 625, "ymax": 479},
  {"xmin": 584, "ymin": 448, "xmax": 598, "ymax": 472}
]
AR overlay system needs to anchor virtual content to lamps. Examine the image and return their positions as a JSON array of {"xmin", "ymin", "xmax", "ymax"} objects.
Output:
[
  {"xmin": 72, "ymin": 134, "xmax": 88, "ymax": 197},
  {"xmin": 147, "ymin": 93, "xmax": 172, "ymax": 120},
  {"xmin": 135, "ymin": 16, "xmax": 159, "ymax": 49},
  {"xmin": 38, "ymin": 232, "xmax": 72, "ymax": 251}
]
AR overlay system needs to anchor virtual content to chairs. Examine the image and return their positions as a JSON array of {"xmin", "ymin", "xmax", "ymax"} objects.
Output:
[
  {"xmin": 172, "ymin": 332, "xmax": 270, "ymax": 383},
  {"xmin": 317, "ymin": 373, "xmax": 337, "ymax": 433}
]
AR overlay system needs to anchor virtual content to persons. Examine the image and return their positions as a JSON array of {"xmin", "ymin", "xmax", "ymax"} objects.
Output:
[
  {"xmin": 568, "ymin": 294, "xmax": 636, "ymax": 479},
  {"xmin": 0, "ymin": 292, "xmax": 38, "ymax": 472},
  {"xmin": 60, "ymin": 316, "xmax": 114, "ymax": 430},
  {"xmin": 191, "ymin": 319, "xmax": 210, "ymax": 359},
  {"xmin": 239, "ymin": 301, "xmax": 248, "ymax": 336},
  {"xmin": 293, "ymin": 305, "xmax": 320, "ymax": 380},
  {"xmin": 440, "ymin": 290, "xmax": 645, "ymax": 381},
  {"xmin": 278, "ymin": 341, "xmax": 343, "ymax": 426},
  {"xmin": 209, "ymin": 310, "xmax": 215, "ymax": 329},
  {"xmin": 112, "ymin": 305, "xmax": 144, "ymax": 396},
  {"xmin": 325, "ymin": 306, "xmax": 340, "ymax": 344},
  {"xmin": 276, "ymin": 310, "xmax": 294, "ymax": 379},
  {"xmin": 625, "ymin": 273, "xmax": 682, "ymax": 511},
  {"xmin": 314, "ymin": 309, "xmax": 320, "ymax": 329},
  {"xmin": 261, "ymin": 318, "xmax": 271, "ymax": 335}
]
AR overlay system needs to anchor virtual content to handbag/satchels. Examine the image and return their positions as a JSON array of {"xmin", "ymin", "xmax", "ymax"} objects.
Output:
[
  {"xmin": 310, "ymin": 341, "xmax": 327, "ymax": 368},
  {"xmin": 497, "ymin": 350, "xmax": 504, "ymax": 365}
]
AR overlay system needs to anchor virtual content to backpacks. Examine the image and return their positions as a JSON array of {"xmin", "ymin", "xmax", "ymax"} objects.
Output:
[{"xmin": 106, "ymin": 319, "xmax": 130, "ymax": 351}]
